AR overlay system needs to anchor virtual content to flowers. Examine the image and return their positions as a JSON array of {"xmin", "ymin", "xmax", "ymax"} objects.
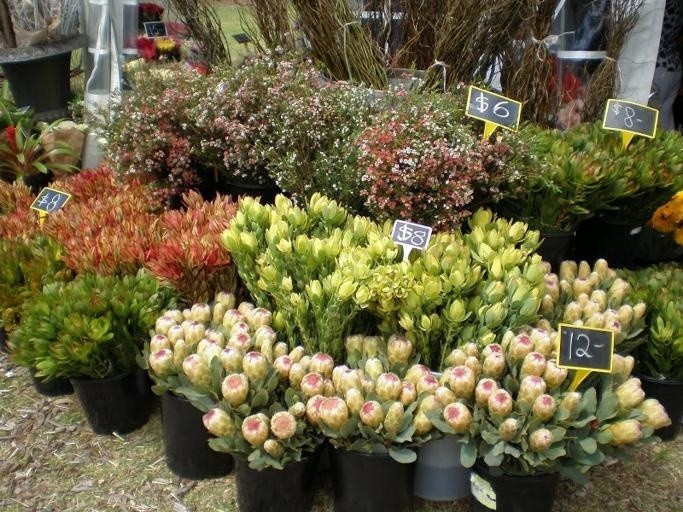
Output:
[{"xmin": 0, "ymin": 4, "xmax": 683, "ymax": 488}]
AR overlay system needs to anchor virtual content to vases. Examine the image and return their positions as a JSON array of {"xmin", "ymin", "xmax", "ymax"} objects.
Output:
[
  {"xmin": 157, "ymin": 390, "xmax": 236, "ymax": 480},
  {"xmin": 632, "ymin": 374, "xmax": 683, "ymax": 440},
  {"xmin": 235, "ymin": 433, "xmax": 561, "ymax": 512},
  {"xmin": 534, "ymin": 214, "xmax": 683, "ymax": 275},
  {"xmin": 30, "ymin": 376, "xmax": 74, "ymax": 397},
  {"xmin": 69, "ymin": 367, "xmax": 153, "ymax": 436}
]
